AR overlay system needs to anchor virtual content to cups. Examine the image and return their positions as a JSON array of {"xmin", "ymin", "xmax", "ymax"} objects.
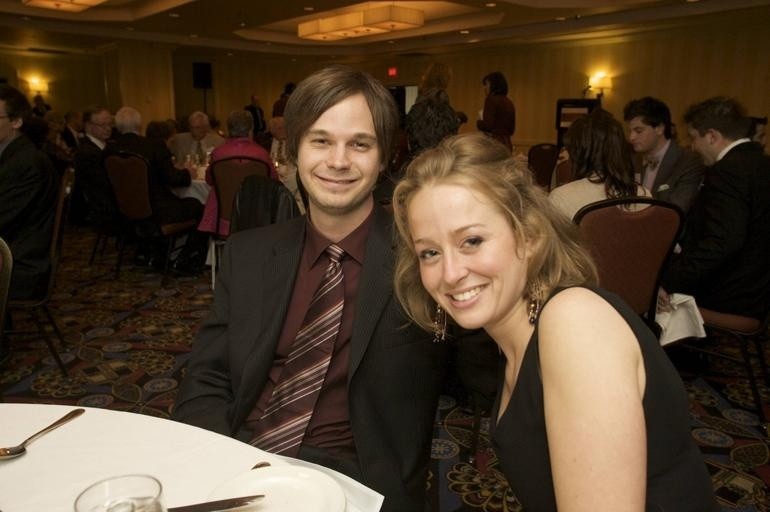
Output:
[{"xmin": 74, "ymin": 473, "xmax": 167, "ymax": 512}]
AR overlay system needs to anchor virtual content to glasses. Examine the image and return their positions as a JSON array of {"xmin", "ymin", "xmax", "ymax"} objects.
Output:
[{"xmin": 90, "ymin": 122, "xmax": 113, "ymax": 127}]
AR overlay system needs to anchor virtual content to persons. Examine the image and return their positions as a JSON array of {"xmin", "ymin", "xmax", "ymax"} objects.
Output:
[
  {"xmin": 393, "ymin": 133, "xmax": 716, "ymax": 512},
  {"xmin": 550, "ymin": 95, "xmax": 770, "ymax": 317},
  {"xmin": 477, "ymin": 72, "xmax": 516, "ymax": 151},
  {"xmin": 0, "ymin": 85, "xmax": 62, "ymax": 299},
  {"xmin": 32, "ymin": 83, "xmax": 305, "ymax": 264},
  {"xmin": 388, "ymin": 63, "xmax": 467, "ymax": 178},
  {"xmin": 175, "ymin": 66, "xmax": 438, "ymax": 512}
]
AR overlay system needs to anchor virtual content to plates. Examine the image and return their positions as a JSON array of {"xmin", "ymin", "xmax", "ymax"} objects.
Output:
[{"xmin": 209, "ymin": 466, "xmax": 348, "ymax": 512}]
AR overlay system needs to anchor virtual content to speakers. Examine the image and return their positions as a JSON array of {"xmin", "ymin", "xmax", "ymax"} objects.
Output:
[{"xmin": 192, "ymin": 63, "xmax": 212, "ymax": 89}]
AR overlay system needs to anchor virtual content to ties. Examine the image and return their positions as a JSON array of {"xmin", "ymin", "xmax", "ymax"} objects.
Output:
[
  {"xmin": 642, "ymin": 154, "xmax": 658, "ymax": 185},
  {"xmin": 247, "ymin": 245, "xmax": 345, "ymax": 459}
]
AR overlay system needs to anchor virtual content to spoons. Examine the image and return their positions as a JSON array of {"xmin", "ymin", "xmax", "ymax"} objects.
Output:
[{"xmin": 0, "ymin": 408, "xmax": 86, "ymax": 460}]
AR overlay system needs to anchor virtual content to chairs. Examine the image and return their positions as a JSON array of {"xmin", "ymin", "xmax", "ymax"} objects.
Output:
[
  {"xmin": 211, "ymin": 155, "xmax": 273, "ymax": 291},
  {"xmin": 571, "ymin": 197, "xmax": 683, "ymax": 340},
  {"xmin": 230, "ymin": 175, "xmax": 302, "ymax": 234},
  {"xmin": 2, "ymin": 167, "xmax": 67, "ymax": 377},
  {"xmin": 661, "ymin": 308, "xmax": 767, "ymax": 423},
  {"xmin": 59, "ymin": 143, "xmax": 198, "ymax": 280},
  {"xmin": 527, "ymin": 145, "xmax": 558, "ymax": 193}
]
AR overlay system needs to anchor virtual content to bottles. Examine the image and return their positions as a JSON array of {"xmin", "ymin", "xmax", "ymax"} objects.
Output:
[{"xmin": 170, "ymin": 152, "xmax": 212, "ymax": 177}]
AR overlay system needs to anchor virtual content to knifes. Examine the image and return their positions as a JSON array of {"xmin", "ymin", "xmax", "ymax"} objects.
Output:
[{"xmin": 165, "ymin": 493, "xmax": 267, "ymax": 512}]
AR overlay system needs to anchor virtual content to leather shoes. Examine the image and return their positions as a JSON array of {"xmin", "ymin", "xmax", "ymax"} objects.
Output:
[{"xmin": 133, "ymin": 257, "xmax": 191, "ymax": 278}]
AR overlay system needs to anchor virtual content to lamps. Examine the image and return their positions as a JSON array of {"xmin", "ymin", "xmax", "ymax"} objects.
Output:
[
  {"xmin": 589, "ymin": 72, "xmax": 612, "ymax": 98},
  {"xmin": 29, "ymin": 76, "xmax": 49, "ymax": 95},
  {"xmin": 297, "ymin": 5, "xmax": 425, "ymax": 42}
]
